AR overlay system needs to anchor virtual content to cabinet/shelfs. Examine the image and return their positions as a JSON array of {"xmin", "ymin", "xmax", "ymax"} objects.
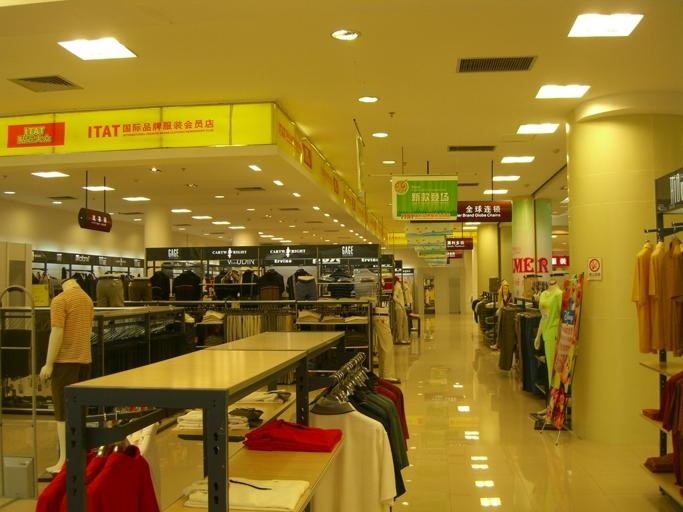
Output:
[
  {"xmin": 0, "ymin": 286, "xmax": 186, "ymax": 499},
  {"xmin": 294, "ymin": 300, "xmax": 372, "ymax": 374},
  {"xmin": 203, "ymin": 331, "xmax": 347, "ymax": 426},
  {"xmin": 186, "ymin": 299, "xmax": 226, "ymax": 350},
  {"xmin": 62, "ymin": 348, "xmax": 345, "ymax": 512},
  {"xmin": 638, "ymin": 360, "xmax": 683, "ymax": 507}
]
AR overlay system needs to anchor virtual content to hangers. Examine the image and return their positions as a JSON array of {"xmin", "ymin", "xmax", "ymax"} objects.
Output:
[
  {"xmin": 328, "ymin": 352, "xmax": 369, "ymax": 404},
  {"xmin": 225, "ymin": 302, "xmax": 294, "ymax": 315}
]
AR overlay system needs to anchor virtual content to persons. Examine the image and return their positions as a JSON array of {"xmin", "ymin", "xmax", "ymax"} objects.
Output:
[
  {"xmin": 375, "ymin": 307, "xmax": 400, "ymax": 383},
  {"xmin": 393, "ymin": 281, "xmax": 412, "ymax": 342},
  {"xmin": 40, "ymin": 278, "xmax": 93, "ymax": 473},
  {"xmin": 534, "ymin": 280, "xmax": 562, "ymax": 414},
  {"xmin": 490, "ymin": 278, "xmax": 510, "ymax": 350}
]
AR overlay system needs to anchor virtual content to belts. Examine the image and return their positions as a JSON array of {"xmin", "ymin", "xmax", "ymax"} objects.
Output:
[
  {"xmin": 372, "ymin": 312, "xmax": 389, "ymax": 317},
  {"xmin": 96, "ymin": 276, "xmax": 122, "ymax": 280}
]
